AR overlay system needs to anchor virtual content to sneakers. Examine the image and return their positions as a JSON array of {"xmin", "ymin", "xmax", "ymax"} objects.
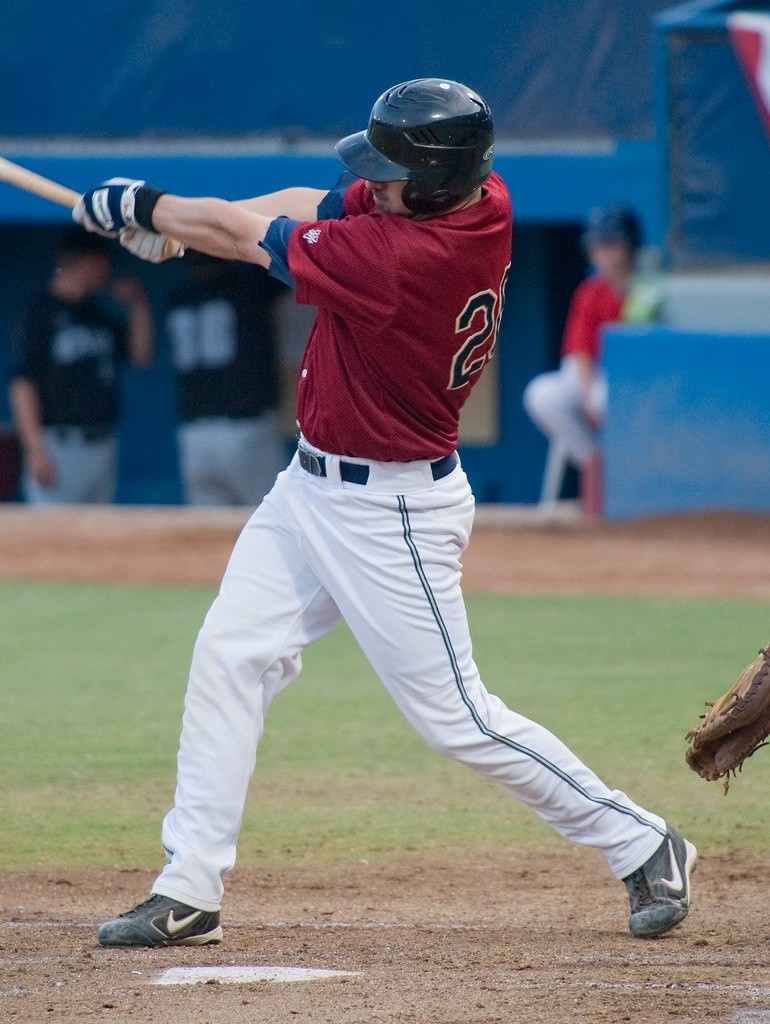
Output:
[
  {"xmin": 622, "ymin": 824, "xmax": 696, "ymax": 939},
  {"xmin": 97, "ymin": 893, "xmax": 224, "ymax": 950}
]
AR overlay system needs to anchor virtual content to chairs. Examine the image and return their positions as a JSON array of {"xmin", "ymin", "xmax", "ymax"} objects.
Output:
[{"xmin": 538, "ymin": 434, "xmax": 603, "ymax": 516}]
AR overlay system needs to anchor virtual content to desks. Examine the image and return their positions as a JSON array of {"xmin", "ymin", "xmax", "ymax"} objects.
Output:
[{"xmin": 598, "ymin": 324, "xmax": 770, "ymax": 520}]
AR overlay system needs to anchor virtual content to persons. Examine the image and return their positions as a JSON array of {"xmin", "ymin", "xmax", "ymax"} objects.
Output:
[
  {"xmin": 12, "ymin": 235, "xmax": 150, "ymax": 504},
  {"xmin": 94, "ymin": 72, "xmax": 699, "ymax": 944}
]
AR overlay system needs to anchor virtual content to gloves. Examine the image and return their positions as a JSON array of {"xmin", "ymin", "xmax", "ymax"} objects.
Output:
[
  {"xmin": 119, "ymin": 227, "xmax": 185, "ymax": 262},
  {"xmin": 72, "ymin": 176, "xmax": 168, "ymax": 238}
]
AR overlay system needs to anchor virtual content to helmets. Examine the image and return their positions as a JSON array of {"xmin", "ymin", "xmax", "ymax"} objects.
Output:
[{"xmin": 335, "ymin": 77, "xmax": 496, "ymax": 215}]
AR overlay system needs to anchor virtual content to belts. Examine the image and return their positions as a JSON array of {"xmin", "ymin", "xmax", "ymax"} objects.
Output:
[{"xmin": 298, "ymin": 445, "xmax": 457, "ymax": 486}]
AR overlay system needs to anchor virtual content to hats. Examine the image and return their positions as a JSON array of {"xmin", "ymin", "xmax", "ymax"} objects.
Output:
[{"xmin": 580, "ymin": 204, "xmax": 644, "ymax": 249}]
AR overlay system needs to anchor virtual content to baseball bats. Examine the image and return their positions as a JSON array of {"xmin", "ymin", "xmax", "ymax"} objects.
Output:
[{"xmin": 0, "ymin": 155, "xmax": 182, "ymax": 259}]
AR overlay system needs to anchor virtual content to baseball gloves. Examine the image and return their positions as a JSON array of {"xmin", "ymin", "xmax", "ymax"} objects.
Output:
[{"xmin": 682, "ymin": 644, "xmax": 770, "ymax": 797}]
